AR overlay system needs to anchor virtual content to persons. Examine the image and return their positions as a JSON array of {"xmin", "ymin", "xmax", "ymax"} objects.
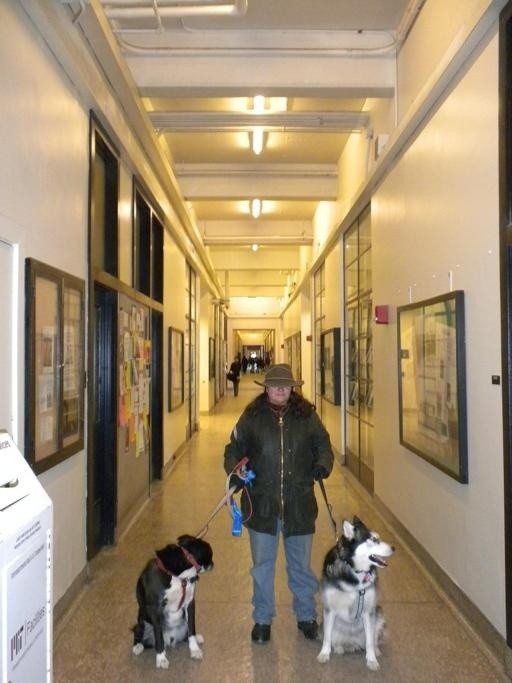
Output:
[
  {"xmin": 224, "ymin": 363, "xmax": 334, "ymax": 644},
  {"xmin": 229, "ymin": 349, "xmax": 266, "ymax": 396}
]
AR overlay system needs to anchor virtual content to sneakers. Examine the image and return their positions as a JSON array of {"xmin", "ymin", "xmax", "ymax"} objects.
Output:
[
  {"xmin": 297, "ymin": 619, "xmax": 320, "ymax": 640},
  {"xmin": 250, "ymin": 621, "xmax": 272, "ymax": 643}
]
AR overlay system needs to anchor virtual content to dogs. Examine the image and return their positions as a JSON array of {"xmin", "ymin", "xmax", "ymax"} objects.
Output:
[
  {"xmin": 314, "ymin": 513, "xmax": 397, "ymax": 672},
  {"xmin": 127, "ymin": 534, "xmax": 215, "ymax": 670}
]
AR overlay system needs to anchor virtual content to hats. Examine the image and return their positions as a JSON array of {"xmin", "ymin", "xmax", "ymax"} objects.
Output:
[{"xmin": 253, "ymin": 362, "xmax": 305, "ymax": 387}]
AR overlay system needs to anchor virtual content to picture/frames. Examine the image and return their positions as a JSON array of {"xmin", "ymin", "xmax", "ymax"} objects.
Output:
[
  {"xmin": 208, "ymin": 336, "xmax": 215, "ymax": 380},
  {"xmin": 23, "ymin": 255, "xmax": 88, "ymax": 476},
  {"xmin": 394, "ymin": 287, "xmax": 470, "ymax": 485},
  {"xmin": 168, "ymin": 325, "xmax": 185, "ymax": 414},
  {"xmin": 320, "ymin": 325, "xmax": 342, "ymax": 408}
]
